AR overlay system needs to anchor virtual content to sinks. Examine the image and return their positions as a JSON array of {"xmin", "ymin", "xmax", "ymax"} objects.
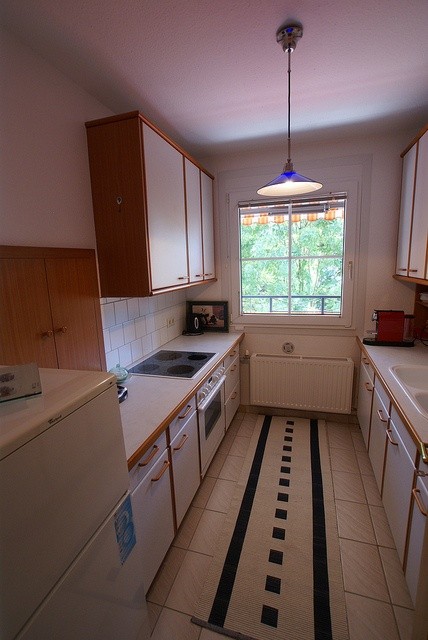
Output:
[{"xmin": 388, "ymin": 364, "xmax": 428, "ymax": 419}]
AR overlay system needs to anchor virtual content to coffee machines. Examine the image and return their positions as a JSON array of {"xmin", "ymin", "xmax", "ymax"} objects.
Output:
[{"xmin": 363, "ymin": 310, "xmax": 417, "ymax": 347}]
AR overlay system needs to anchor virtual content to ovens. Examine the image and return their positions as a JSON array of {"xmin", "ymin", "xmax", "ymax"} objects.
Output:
[{"xmin": 199, "ymin": 375, "xmax": 226, "ymax": 467}]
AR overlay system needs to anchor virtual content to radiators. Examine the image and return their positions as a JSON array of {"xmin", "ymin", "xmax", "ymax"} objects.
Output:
[{"xmin": 250, "ymin": 352, "xmax": 355, "ymax": 415}]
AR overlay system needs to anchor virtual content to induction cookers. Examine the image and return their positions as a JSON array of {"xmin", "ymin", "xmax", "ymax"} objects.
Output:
[{"xmin": 128, "ymin": 349, "xmax": 216, "ymax": 379}]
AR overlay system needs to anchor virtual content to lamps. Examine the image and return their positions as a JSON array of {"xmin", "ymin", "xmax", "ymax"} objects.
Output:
[{"xmin": 256, "ymin": 25, "xmax": 323, "ymax": 196}]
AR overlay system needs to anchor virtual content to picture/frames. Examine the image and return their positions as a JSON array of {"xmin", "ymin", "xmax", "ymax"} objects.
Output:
[{"xmin": 185, "ymin": 300, "xmax": 229, "ymax": 334}]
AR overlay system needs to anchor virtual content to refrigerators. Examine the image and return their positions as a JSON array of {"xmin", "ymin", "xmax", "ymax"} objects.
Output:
[{"xmin": 0, "ymin": 369, "xmax": 152, "ymax": 640}]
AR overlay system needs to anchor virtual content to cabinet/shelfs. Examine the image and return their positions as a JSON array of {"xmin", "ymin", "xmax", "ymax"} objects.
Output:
[
  {"xmin": 183, "ymin": 156, "xmax": 217, "ymax": 284},
  {"xmin": 403, "ymin": 443, "xmax": 428, "ymax": 608},
  {"xmin": 224, "ymin": 343, "xmax": 241, "ymax": 432},
  {"xmin": 368, "ymin": 372, "xmax": 419, "ymax": 570},
  {"xmin": 0, "ymin": 246, "xmax": 107, "ymax": 371},
  {"xmin": 356, "ymin": 349, "xmax": 375, "ymax": 452},
  {"xmin": 392, "ymin": 123, "xmax": 428, "ymax": 286},
  {"xmin": 126, "ymin": 394, "xmax": 202, "ymax": 597},
  {"xmin": 84, "ymin": 109, "xmax": 189, "ymax": 295}
]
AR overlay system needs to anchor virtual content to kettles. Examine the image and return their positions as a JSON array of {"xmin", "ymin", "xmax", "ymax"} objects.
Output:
[{"xmin": 187, "ymin": 312, "xmax": 208, "ymax": 336}]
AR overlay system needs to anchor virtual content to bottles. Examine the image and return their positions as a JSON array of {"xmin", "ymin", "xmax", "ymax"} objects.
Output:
[{"xmin": 107, "ymin": 364, "xmax": 128, "ymax": 382}]
